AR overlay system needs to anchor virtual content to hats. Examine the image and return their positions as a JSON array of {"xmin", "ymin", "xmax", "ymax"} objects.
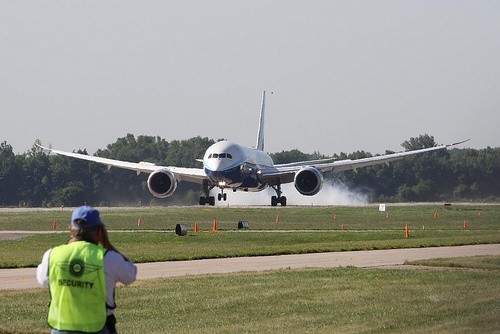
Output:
[{"xmin": 70, "ymin": 206, "xmax": 106, "ymax": 226}]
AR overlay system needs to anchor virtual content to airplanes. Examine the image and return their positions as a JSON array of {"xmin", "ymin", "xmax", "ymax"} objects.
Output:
[{"xmin": 36, "ymin": 90, "xmax": 471, "ymax": 206}]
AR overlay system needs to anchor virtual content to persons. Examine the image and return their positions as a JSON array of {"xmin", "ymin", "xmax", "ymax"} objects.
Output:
[{"xmin": 37, "ymin": 205, "xmax": 137, "ymax": 334}]
